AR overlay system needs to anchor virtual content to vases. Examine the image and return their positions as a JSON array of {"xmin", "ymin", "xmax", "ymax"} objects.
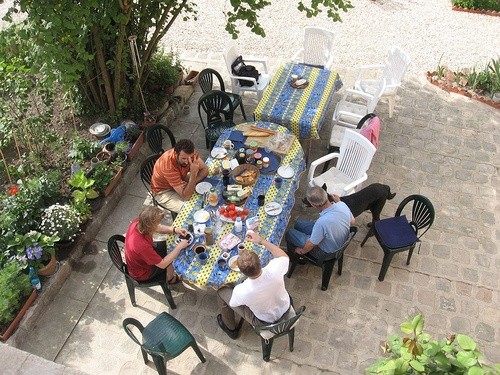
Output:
[{"xmin": 37, "ymin": 248, "xmax": 56, "ymax": 276}]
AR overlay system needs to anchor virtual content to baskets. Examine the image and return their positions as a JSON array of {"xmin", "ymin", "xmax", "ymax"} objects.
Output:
[
  {"xmin": 231, "ymin": 163, "xmax": 261, "ymax": 187},
  {"xmin": 221, "ymin": 188, "xmax": 249, "ymax": 207}
]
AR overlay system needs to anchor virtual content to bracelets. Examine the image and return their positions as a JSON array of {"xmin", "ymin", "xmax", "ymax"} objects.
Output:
[
  {"xmin": 258, "ymin": 238, "xmax": 264, "ymax": 245},
  {"xmin": 173, "ymin": 227, "xmax": 177, "ymax": 234}
]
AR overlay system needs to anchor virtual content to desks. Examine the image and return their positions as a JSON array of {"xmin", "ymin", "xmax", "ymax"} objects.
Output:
[
  {"xmin": 251, "ymin": 63, "xmax": 343, "ymax": 167},
  {"xmin": 165, "ymin": 121, "xmax": 307, "ymax": 292}
]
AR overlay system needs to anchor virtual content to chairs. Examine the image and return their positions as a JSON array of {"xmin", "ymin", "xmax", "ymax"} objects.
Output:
[
  {"xmin": 359, "ymin": 50, "xmax": 411, "ymax": 118},
  {"xmin": 288, "ymin": 227, "xmax": 358, "ymax": 291},
  {"xmin": 146, "ymin": 124, "xmax": 176, "ymax": 154},
  {"xmin": 199, "ymin": 43, "xmax": 272, "ymax": 153},
  {"xmin": 236, "ymin": 306, "xmax": 306, "ymax": 362},
  {"xmin": 322, "ymin": 114, "xmax": 380, "ymax": 175},
  {"xmin": 306, "ymin": 128, "xmax": 377, "ymax": 195},
  {"xmin": 122, "ymin": 312, "xmax": 206, "ymax": 375},
  {"xmin": 108, "ymin": 234, "xmax": 176, "ymax": 310},
  {"xmin": 292, "ymin": 27, "xmax": 336, "ymax": 69},
  {"xmin": 333, "ymin": 79, "xmax": 386, "ymax": 129},
  {"xmin": 140, "ymin": 156, "xmax": 178, "ymax": 222},
  {"xmin": 361, "ymin": 195, "xmax": 435, "ymax": 282}
]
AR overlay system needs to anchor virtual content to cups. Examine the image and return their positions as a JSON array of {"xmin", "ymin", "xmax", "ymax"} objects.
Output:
[
  {"xmin": 238, "ymin": 244, "xmax": 246, "ymax": 255},
  {"xmin": 198, "ymin": 253, "xmax": 207, "ymax": 265},
  {"xmin": 224, "ymin": 140, "xmax": 234, "ymax": 150},
  {"xmin": 222, "ymin": 169, "xmax": 229, "ymax": 186},
  {"xmin": 218, "ymin": 259, "xmax": 227, "ymax": 271}
]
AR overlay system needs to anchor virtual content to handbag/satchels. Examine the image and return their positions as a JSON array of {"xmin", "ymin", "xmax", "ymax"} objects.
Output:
[{"xmin": 232, "ymin": 57, "xmax": 261, "ymax": 87}]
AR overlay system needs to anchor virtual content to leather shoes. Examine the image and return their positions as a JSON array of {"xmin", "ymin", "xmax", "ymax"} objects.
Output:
[{"xmin": 217, "ymin": 313, "xmax": 238, "ymax": 339}]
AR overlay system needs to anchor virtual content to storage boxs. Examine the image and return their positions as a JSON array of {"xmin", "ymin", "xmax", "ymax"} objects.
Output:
[{"xmin": 185, "ymin": 70, "xmax": 199, "ymax": 88}]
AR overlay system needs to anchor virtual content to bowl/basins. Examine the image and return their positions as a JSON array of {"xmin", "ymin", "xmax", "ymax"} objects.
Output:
[
  {"xmin": 231, "ymin": 164, "xmax": 259, "ymax": 186},
  {"xmin": 192, "ymin": 244, "xmax": 206, "ymax": 255}
]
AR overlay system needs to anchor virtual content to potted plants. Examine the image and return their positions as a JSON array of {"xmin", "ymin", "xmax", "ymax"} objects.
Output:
[{"xmin": 0, "ymin": 44, "xmax": 184, "ymax": 340}]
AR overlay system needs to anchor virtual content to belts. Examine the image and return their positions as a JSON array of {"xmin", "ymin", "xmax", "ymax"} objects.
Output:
[{"xmin": 259, "ymin": 305, "xmax": 290, "ymax": 326}]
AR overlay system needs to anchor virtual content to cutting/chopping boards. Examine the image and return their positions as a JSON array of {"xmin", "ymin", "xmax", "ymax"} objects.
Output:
[{"xmin": 245, "ymin": 133, "xmax": 296, "ymax": 155}]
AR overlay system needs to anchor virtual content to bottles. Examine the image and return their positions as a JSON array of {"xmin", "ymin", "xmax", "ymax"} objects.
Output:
[
  {"xmin": 209, "ymin": 187, "xmax": 218, "ymax": 208},
  {"xmin": 234, "ymin": 217, "xmax": 242, "ymax": 232},
  {"xmin": 239, "ymin": 142, "xmax": 270, "ymax": 169}
]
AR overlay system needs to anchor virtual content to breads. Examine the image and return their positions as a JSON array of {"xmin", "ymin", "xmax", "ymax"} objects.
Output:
[
  {"xmin": 249, "ymin": 125, "xmax": 276, "ymax": 134},
  {"xmin": 242, "ymin": 132, "xmax": 270, "ymax": 137},
  {"xmin": 236, "ymin": 171, "xmax": 256, "ymax": 182}
]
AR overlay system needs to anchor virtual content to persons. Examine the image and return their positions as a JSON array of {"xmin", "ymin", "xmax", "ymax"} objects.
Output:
[
  {"xmin": 217, "ymin": 229, "xmax": 291, "ymax": 340},
  {"xmin": 150, "ymin": 139, "xmax": 209, "ymax": 213},
  {"xmin": 125, "ymin": 206, "xmax": 190, "ymax": 285},
  {"xmin": 280, "ymin": 184, "xmax": 355, "ymax": 260}
]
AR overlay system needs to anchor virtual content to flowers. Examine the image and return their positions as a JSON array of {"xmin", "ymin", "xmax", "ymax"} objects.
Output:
[
  {"xmin": 38, "ymin": 203, "xmax": 86, "ymax": 240},
  {"xmin": 10, "ymin": 187, "xmax": 18, "ymax": 194},
  {"xmin": 13, "ymin": 230, "xmax": 61, "ymax": 270}
]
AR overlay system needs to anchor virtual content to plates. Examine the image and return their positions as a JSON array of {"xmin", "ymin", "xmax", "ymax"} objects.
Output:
[
  {"xmin": 216, "ymin": 206, "xmax": 247, "ymax": 223},
  {"xmin": 193, "ymin": 210, "xmax": 210, "ymax": 223},
  {"xmin": 229, "ymin": 255, "xmax": 241, "ymax": 271},
  {"xmin": 289, "ymin": 78, "xmax": 309, "ymax": 89},
  {"xmin": 264, "ymin": 202, "xmax": 282, "ymax": 216},
  {"xmin": 222, "ymin": 188, "xmax": 248, "ymax": 206},
  {"xmin": 211, "ymin": 147, "xmax": 228, "ymax": 159},
  {"xmin": 174, "ymin": 232, "xmax": 194, "ymax": 246},
  {"xmin": 277, "ymin": 166, "xmax": 294, "ymax": 178},
  {"xmin": 195, "ymin": 181, "xmax": 213, "ymax": 195}
]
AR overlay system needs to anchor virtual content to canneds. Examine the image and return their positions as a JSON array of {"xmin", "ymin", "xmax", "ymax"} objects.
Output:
[{"xmin": 238, "ymin": 142, "xmax": 270, "ymax": 169}]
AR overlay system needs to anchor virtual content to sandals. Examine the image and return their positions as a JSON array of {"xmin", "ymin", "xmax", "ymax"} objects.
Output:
[{"xmin": 166, "ymin": 273, "xmax": 182, "ymax": 284}]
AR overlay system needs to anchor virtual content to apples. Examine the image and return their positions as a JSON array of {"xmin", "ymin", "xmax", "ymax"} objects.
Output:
[{"xmin": 218, "ymin": 205, "xmax": 248, "ymax": 221}]
